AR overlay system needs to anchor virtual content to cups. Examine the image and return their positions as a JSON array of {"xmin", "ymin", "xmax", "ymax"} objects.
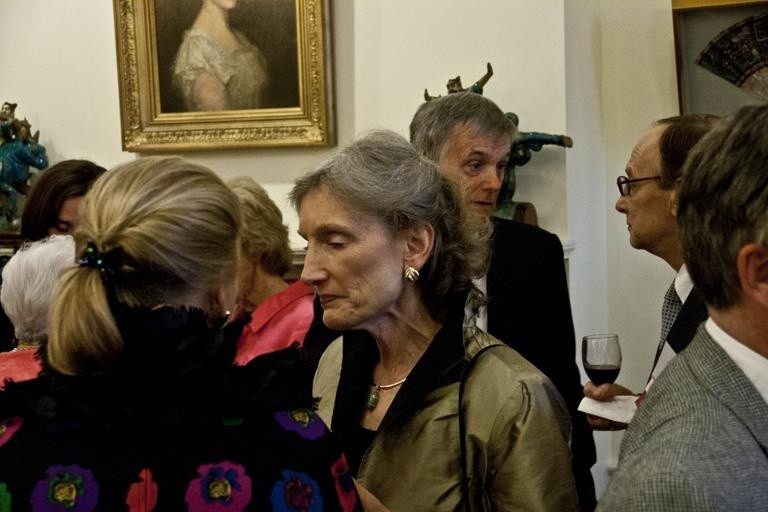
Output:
[{"xmin": 581, "ymin": 334, "xmax": 623, "ymax": 384}]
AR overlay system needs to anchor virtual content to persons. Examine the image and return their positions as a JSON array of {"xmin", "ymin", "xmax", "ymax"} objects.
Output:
[
  {"xmin": 164, "ymin": 0, "xmax": 269, "ymax": 115},
  {"xmin": 217, "ymin": 175, "xmax": 315, "ymax": 366},
  {"xmin": 408, "ymin": 93, "xmax": 601, "ymax": 512},
  {"xmin": 576, "ymin": 112, "xmax": 728, "ymax": 436},
  {"xmin": 597, "ymin": 102, "xmax": 768, "ymax": 511},
  {"xmin": 296, "ymin": 129, "xmax": 583, "ymax": 512},
  {"xmin": 1, "ymin": 232, "xmax": 76, "ymax": 391},
  {"xmin": 14, "ymin": 160, "xmax": 108, "ymax": 245},
  {"xmin": 0, "ymin": 154, "xmax": 366, "ymax": 511}
]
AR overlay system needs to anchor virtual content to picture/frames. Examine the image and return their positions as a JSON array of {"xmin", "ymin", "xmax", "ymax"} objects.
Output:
[{"xmin": 112, "ymin": 1, "xmax": 338, "ymax": 151}]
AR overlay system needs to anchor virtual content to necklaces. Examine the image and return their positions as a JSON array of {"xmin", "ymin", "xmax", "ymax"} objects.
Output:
[{"xmin": 362, "ymin": 370, "xmax": 413, "ymax": 414}]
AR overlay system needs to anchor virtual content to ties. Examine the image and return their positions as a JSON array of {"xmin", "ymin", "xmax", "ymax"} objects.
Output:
[{"xmin": 658, "ymin": 280, "xmax": 682, "ymax": 352}]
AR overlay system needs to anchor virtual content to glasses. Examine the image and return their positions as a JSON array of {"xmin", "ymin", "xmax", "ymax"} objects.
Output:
[{"xmin": 617, "ymin": 175, "xmax": 670, "ymax": 195}]
